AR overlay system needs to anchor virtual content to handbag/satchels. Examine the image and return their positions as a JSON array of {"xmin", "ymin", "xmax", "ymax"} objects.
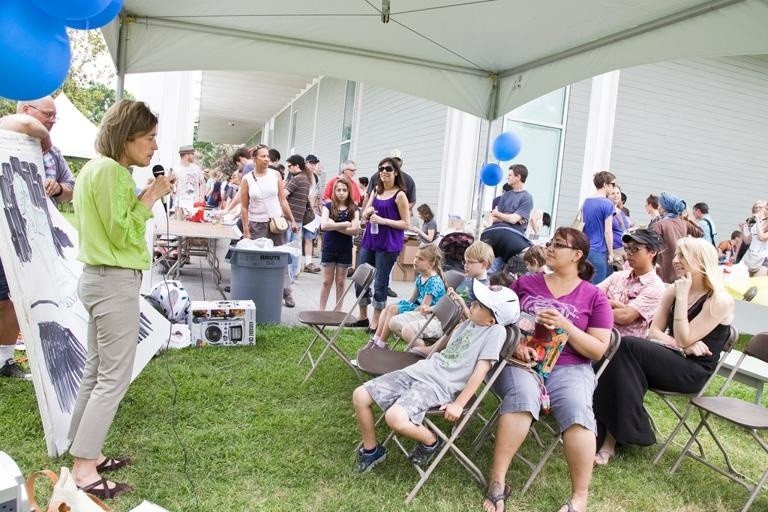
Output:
[{"xmin": 268, "ymin": 216, "xmax": 289, "ymax": 234}]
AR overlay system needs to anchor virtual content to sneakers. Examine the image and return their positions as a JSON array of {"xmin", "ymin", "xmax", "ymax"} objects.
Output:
[
  {"xmin": 359, "ymin": 338, "xmax": 374, "ymax": 350},
  {"xmin": 408, "ymin": 431, "xmax": 444, "ymax": 465},
  {"xmin": 368, "ymin": 342, "xmax": 388, "ymax": 350},
  {"xmin": 354, "ymin": 445, "xmax": 389, "ymax": 473},
  {"xmin": 283, "ymin": 292, "xmax": 295, "ymax": 307},
  {"xmin": 366, "ymin": 324, "xmax": 378, "ymax": 335},
  {"xmin": 0, "ymin": 357, "xmax": 33, "ymax": 382},
  {"xmin": 345, "ymin": 318, "xmax": 369, "ymax": 327}
]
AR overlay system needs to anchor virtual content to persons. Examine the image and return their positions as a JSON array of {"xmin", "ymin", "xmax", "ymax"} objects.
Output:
[
  {"xmin": 738, "ymin": 201, "xmax": 768, "ymax": 277},
  {"xmin": 409, "ymin": 203, "xmax": 438, "ymax": 244},
  {"xmin": 169, "ymin": 144, "xmax": 205, "ymax": 264},
  {"xmin": 593, "ymin": 235, "xmax": 736, "ymax": 467},
  {"xmin": 605, "ymin": 184, "xmax": 632, "ymax": 270},
  {"xmin": 578, "ymin": 171, "xmax": 616, "ymax": 285},
  {"xmin": 491, "ymin": 164, "xmax": 534, "ymax": 235},
  {"xmin": 268, "ymin": 148, "xmax": 285, "ymax": 180},
  {"xmin": 346, "ymin": 157, "xmax": 411, "ymax": 335},
  {"xmin": 648, "ymin": 192, "xmax": 705, "ymax": 284},
  {"xmin": 595, "ymin": 225, "xmax": 665, "ymax": 340},
  {"xmin": 617, "ymin": 192, "xmax": 630, "ymax": 216},
  {"xmin": 492, "ymin": 182, "xmax": 513, "ymax": 211},
  {"xmin": 388, "ymin": 240, "xmax": 496, "ymax": 348},
  {"xmin": 692, "ymin": 202, "xmax": 718, "ymax": 248},
  {"xmin": 644, "ymin": 195, "xmax": 664, "ymax": 228},
  {"xmin": 322, "ymin": 159, "xmax": 361, "ymax": 278},
  {"xmin": 225, "ymin": 170, "xmax": 240, "ymax": 209},
  {"xmin": 302, "ymin": 154, "xmax": 321, "ymax": 274},
  {"xmin": 282, "ymin": 154, "xmax": 312, "ymax": 284},
  {"xmin": 66, "ymin": 98, "xmax": 174, "ymax": 501},
  {"xmin": 718, "ymin": 230, "xmax": 742, "ymax": 255},
  {"xmin": 481, "ymin": 226, "xmax": 614, "ymax": 512},
  {"xmin": 215, "ymin": 146, "xmax": 255, "ymax": 293},
  {"xmin": 0, "ymin": 95, "xmax": 76, "ymax": 381},
  {"xmin": 363, "ymin": 242, "xmax": 448, "ymax": 351},
  {"xmin": 239, "ymin": 145, "xmax": 299, "ymax": 308},
  {"xmin": 523, "ymin": 243, "xmax": 550, "ymax": 274},
  {"xmin": 359, "ymin": 148, "xmax": 417, "ymax": 298},
  {"xmin": 205, "ymin": 170, "xmax": 220, "ymax": 207},
  {"xmin": 358, "ymin": 176, "xmax": 369, "ymax": 197},
  {"xmin": 736, "ymin": 200, "xmax": 766, "ymax": 263},
  {"xmin": 319, "ymin": 177, "xmax": 361, "ymax": 312},
  {"xmin": 351, "ymin": 277, "xmax": 521, "ymax": 475}
]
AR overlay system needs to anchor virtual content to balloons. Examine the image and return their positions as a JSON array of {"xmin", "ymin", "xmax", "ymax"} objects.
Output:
[
  {"xmin": 0, "ymin": 0, "xmax": 72, "ymax": 101},
  {"xmin": 480, "ymin": 163, "xmax": 504, "ymax": 186},
  {"xmin": 31, "ymin": 0, "xmax": 124, "ymax": 30},
  {"xmin": 492, "ymin": 131, "xmax": 522, "ymax": 161}
]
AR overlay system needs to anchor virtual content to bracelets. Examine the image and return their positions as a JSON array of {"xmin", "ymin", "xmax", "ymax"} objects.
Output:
[{"xmin": 53, "ymin": 182, "xmax": 64, "ymax": 197}]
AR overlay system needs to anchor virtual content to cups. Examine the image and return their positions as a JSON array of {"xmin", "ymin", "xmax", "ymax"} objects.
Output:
[{"xmin": 533, "ymin": 302, "xmax": 550, "ymax": 324}]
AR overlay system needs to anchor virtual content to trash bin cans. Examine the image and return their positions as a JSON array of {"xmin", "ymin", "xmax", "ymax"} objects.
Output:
[{"xmin": 225, "ymin": 243, "xmax": 289, "ymax": 326}]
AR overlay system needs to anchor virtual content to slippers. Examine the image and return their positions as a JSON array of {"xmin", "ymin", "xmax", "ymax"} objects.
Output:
[
  {"xmin": 556, "ymin": 498, "xmax": 582, "ymax": 512},
  {"xmin": 483, "ymin": 484, "xmax": 511, "ymax": 512}
]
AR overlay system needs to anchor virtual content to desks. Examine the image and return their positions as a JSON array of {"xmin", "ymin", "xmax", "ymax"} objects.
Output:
[{"xmin": 154, "ymin": 218, "xmax": 239, "ymax": 301}]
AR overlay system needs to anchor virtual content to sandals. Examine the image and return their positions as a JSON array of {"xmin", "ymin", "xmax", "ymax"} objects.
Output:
[
  {"xmin": 74, "ymin": 478, "xmax": 130, "ymax": 499},
  {"xmin": 96, "ymin": 455, "xmax": 131, "ymax": 473}
]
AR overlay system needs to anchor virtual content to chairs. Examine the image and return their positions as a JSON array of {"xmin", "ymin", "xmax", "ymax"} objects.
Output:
[
  {"xmin": 353, "ymin": 293, "xmax": 465, "ymax": 453},
  {"xmin": 648, "ymin": 324, "xmax": 740, "ymax": 466},
  {"xmin": 472, "ymin": 326, "xmax": 622, "ymax": 495},
  {"xmin": 670, "ymin": 330, "xmax": 768, "ymax": 512},
  {"xmin": 382, "ymin": 323, "xmax": 521, "ymax": 506},
  {"xmin": 144, "ymin": 218, "xmax": 178, "ymax": 276},
  {"xmin": 296, "ymin": 262, "xmax": 377, "ymax": 388},
  {"xmin": 444, "ymin": 270, "xmax": 465, "ymax": 291}
]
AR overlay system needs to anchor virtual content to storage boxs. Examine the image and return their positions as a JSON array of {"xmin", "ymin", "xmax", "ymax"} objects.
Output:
[{"xmin": 399, "ymin": 240, "xmax": 419, "ymax": 265}]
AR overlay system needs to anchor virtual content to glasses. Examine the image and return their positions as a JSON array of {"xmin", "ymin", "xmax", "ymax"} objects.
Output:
[
  {"xmin": 28, "ymin": 105, "xmax": 57, "ymax": 119},
  {"xmin": 378, "ymin": 166, "xmax": 393, "ymax": 172},
  {"xmin": 624, "ymin": 246, "xmax": 642, "ymax": 253},
  {"xmin": 288, "ymin": 165, "xmax": 292, "ymax": 168},
  {"xmin": 610, "ymin": 182, "xmax": 616, "ymax": 187},
  {"xmin": 546, "ymin": 241, "xmax": 575, "ymax": 251},
  {"xmin": 461, "ymin": 261, "xmax": 479, "ymax": 265}
]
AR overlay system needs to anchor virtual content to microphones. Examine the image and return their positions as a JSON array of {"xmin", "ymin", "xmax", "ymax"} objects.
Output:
[{"xmin": 152, "ymin": 164, "xmax": 169, "ymax": 215}]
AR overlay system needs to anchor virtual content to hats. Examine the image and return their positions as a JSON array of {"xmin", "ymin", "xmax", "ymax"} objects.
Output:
[
  {"xmin": 473, "ymin": 277, "xmax": 521, "ymax": 327},
  {"xmin": 622, "ymin": 228, "xmax": 662, "ymax": 250},
  {"xmin": 179, "ymin": 145, "xmax": 195, "ymax": 154},
  {"xmin": 659, "ymin": 192, "xmax": 684, "ymax": 214},
  {"xmin": 305, "ymin": 155, "xmax": 319, "ymax": 162}
]
